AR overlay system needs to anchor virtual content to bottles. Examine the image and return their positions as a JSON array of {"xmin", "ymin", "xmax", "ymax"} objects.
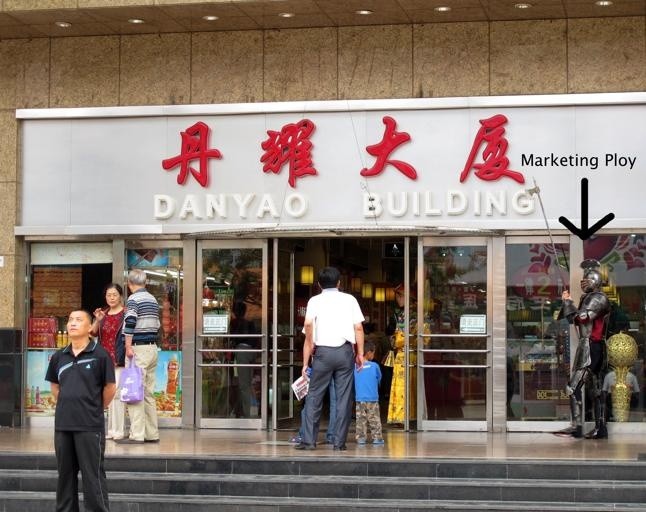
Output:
[
  {"xmin": 63, "ymin": 331, "xmax": 67, "ymax": 348},
  {"xmin": 26, "ymin": 384, "xmax": 41, "ymax": 408},
  {"xmin": 57, "ymin": 330, "xmax": 62, "ymax": 348},
  {"xmin": 167, "ymin": 354, "xmax": 180, "ymax": 394}
]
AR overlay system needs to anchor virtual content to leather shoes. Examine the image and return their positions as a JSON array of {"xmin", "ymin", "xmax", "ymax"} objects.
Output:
[
  {"xmin": 293, "ymin": 442, "xmax": 317, "ymax": 451},
  {"xmin": 333, "ymin": 445, "xmax": 347, "ymax": 452}
]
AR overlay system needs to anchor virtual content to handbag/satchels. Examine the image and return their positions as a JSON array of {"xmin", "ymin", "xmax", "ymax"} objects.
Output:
[{"xmin": 119, "ymin": 354, "xmax": 145, "ymax": 404}]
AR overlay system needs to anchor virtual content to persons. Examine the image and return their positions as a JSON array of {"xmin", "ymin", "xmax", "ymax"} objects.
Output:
[
  {"xmin": 44, "ymin": 310, "xmax": 115, "ymax": 511},
  {"xmin": 352, "ymin": 341, "xmax": 384, "ymax": 444},
  {"xmin": 387, "ymin": 285, "xmax": 430, "ymax": 429},
  {"xmin": 116, "ymin": 268, "xmax": 161, "ymax": 444},
  {"xmin": 288, "ymin": 323, "xmax": 357, "ymax": 445},
  {"xmin": 90, "ymin": 283, "xmax": 127, "ymax": 441},
  {"xmin": 294, "ymin": 268, "xmax": 365, "ymax": 450},
  {"xmin": 223, "ymin": 301, "xmax": 258, "ymax": 419},
  {"xmin": 553, "ymin": 259, "xmax": 611, "ymax": 439},
  {"xmin": 424, "ymin": 299, "xmax": 645, "ymax": 420}
]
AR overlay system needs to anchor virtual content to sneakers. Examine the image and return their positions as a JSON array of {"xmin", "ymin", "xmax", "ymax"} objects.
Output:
[
  {"xmin": 357, "ymin": 439, "xmax": 368, "ymax": 445},
  {"xmin": 372, "ymin": 437, "xmax": 385, "ymax": 444},
  {"xmin": 553, "ymin": 424, "xmax": 609, "ymax": 440},
  {"xmin": 105, "ymin": 434, "xmax": 160, "ymax": 445}
]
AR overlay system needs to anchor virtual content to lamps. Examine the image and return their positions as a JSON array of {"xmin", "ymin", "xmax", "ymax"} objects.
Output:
[{"xmin": 302, "ymin": 264, "xmax": 395, "ymax": 304}]
